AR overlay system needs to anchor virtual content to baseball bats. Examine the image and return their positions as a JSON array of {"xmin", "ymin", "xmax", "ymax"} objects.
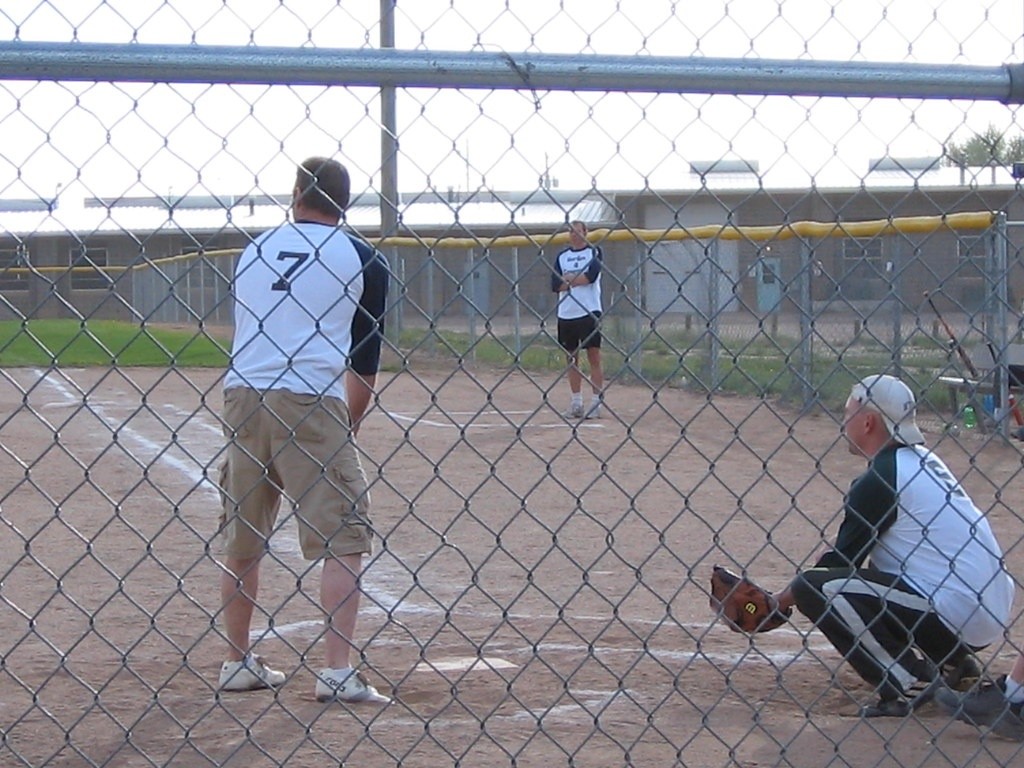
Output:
[
  {"xmin": 987, "ymin": 339, "xmax": 1024, "ymax": 427},
  {"xmin": 920, "ymin": 289, "xmax": 983, "ymax": 382},
  {"xmin": 946, "ymin": 339, "xmax": 988, "ymax": 435}
]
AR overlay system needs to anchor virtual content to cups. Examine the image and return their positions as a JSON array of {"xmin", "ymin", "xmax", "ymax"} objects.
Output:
[
  {"xmin": 994, "ymin": 408, "xmax": 1011, "ymax": 427},
  {"xmin": 983, "ymin": 394, "xmax": 993, "ymax": 420}
]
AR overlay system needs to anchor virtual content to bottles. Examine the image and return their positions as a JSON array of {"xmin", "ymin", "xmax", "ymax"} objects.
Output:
[{"xmin": 964, "ymin": 404, "xmax": 975, "ymax": 429}]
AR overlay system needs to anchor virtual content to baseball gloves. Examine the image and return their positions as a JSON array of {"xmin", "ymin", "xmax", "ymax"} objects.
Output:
[{"xmin": 709, "ymin": 564, "xmax": 792, "ymax": 632}]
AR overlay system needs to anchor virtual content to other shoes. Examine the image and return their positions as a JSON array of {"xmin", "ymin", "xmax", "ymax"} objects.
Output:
[
  {"xmin": 571, "ymin": 405, "xmax": 584, "ymax": 417},
  {"xmin": 584, "ymin": 405, "xmax": 601, "ymax": 419}
]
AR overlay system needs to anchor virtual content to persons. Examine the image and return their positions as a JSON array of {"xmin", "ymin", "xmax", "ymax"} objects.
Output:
[
  {"xmin": 219, "ymin": 156, "xmax": 397, "ymax": 705},
  {"xmin": 709, "ymin": 374, "xmax": 1015, "ymax": 718},
  {"xmin": 550, "ymin": 220, "xmax": 604, "ymax": 419},
  {"xmin": 935, "ymin": 643, "xmax": 1024, "ymax": 741}
]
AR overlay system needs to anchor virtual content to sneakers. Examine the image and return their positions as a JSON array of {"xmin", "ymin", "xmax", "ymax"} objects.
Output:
[
  {"xmin": 925, "ymin": 652, "xmax": 989, "ymax": 694},
  {"xmin": 857, "ymin": 662, "xmax": 941, "ymax": 716},
  {"xmin": 220, "ymin": 652, "xmax": 285, "ymax": 690},
  {"xmin": 315, "ymin": 668, "xmax": 394, "ymax": 704},
  {"xmin": 931, "ymin": 674, "xmax": 1024, "ymax": 742}
]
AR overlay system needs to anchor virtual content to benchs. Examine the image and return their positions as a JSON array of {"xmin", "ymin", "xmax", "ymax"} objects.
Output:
[{"xmin": 939, "ymin": 344, "xmax": 1024, "ymax": 434}]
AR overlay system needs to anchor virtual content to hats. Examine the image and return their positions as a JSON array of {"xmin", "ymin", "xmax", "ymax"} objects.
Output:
[{"xmin": 850, "ymin": 374, "xmax": 926, "ymax": 446}]
